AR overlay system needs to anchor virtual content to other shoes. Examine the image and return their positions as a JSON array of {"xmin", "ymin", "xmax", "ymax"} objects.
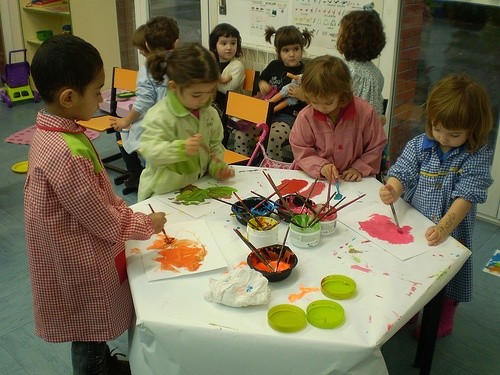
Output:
[{"xmin": 98, "ymin": 345, "xmax": 131, "ymax": 375}]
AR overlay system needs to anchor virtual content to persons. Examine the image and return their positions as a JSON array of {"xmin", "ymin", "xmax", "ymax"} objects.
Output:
[
  {"xmin": 264, "ymin": 70, "xmax": 304, "ymax": 112},
  {"xmin": 136, "ymin": 42, "xmax": 236, "ymax": 202},
  {"xmin": 24, "ymin": 34, "xmax": 167, "ymax": 375},
  {"xmin": 378, "ymin": 71, "xmax": 494, "ymax": 338},
  {"xmin": 109, "ymin": 16, "xmax": 179, "ymax": 174},
  {"xmin": 287, "ymin": 10, "xmax": 386, "ymax": 125},
  {"xmin": 288, "ymin": 54, "xmax": 388, "ymax": 182},
  {"xmin": 209, "ymin": 23, "xmax": 246, "ymax": 149},
  {"xmin": 258, "ymin": 26, "xmax": 314, "ymax": 157}
]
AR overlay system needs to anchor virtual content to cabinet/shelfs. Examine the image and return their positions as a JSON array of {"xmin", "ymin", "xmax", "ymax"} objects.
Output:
[{"xmin": 18, "ymin": 0, "xmax": 121, "ymax": 95}]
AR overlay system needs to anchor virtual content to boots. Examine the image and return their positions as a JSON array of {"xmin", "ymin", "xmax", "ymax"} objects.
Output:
[
  {"xmin": 399, "ymin": 311, "xmax": 420, "ymax": 334},
  {"xmin": 414, "ymin": 298, "xmax": 458, "ymax": 340}
]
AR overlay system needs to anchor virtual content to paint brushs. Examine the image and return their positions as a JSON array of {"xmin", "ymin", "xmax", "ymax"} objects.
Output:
[
  {"xmin": 211, "ymin": 197, "xmax": 245, "ymax": 208},
  {"xmin": 233, "ymin": 192, "xmax": 263, "ymax": 228},
  {"xmin": 327, "ymin": 164, "xmax": 332, "ymax": 208},
  {"xmin": 308, "ymin": 192, "xmax": 336, "ymax": 225},
  {"xmin": 251, "ymin": 191, "xmax": 286, "ymax": 209},
  {"xmin": 262, "ymin": 170, "xmax": 293, "ymax": 212},
  {"xmin": 299, "ymin": 178, "xmax": 317, "ymax": 214},
  {"xmin": 148, "ymin": 203, "xmax": 169, "ymax": 241},
  {"xmin": 251, "ymin": 183, "xmax": 288, "ymax": 212},
  {"xmin": 232, "ymin": 228, "xmax": 274, "ymax": 270},
  {"xmin": 229, "ymin": 213, "xmax": 249, "ymax": 215},
  {"xmin": 329, "ymin": 194, "xmax": 366, "ymax": 215},
  {"xmin": 380, "ymin": 172, "xmax": 403, "ymax": 233},
  {"xmin": 295, "ymin": 191, "xmax": 317, "ymax": 215},
  {"xmin": 274, "ymin": 226, "xmax": 289, "ymax": 271},
  {"xmin": 266, "ymin": 217, "xmax": 287, "ymax": 229},
  {"xmin": 310, "ymin": 196, "xmax": 346, "ymax": 226}
]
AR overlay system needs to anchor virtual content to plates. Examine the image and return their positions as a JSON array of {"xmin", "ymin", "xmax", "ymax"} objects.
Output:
[{"xmin": 117, "ymin": 91, "xmax": 135, "ymax": 98}]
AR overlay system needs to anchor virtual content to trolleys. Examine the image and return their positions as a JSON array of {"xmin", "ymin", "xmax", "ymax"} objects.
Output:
[{"xmin": 0, "ymin": 48, "xmax": 41, "ymax": 109}]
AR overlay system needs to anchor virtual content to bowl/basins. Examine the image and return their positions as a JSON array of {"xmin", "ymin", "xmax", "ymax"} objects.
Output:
[
  {"xmin": 231, "ymin": 197, "xmax": 275, "ymax": 225},
  {"xmin": 247, "ymin": 244, "xmax": 298, "ymax": 282},
  {"xmin": 275, "ymin": 193, "xmax": 313, "ymax": 220},
  {"xmin": 36, "ymin": 30, "xmax": 53, "ymax": 41}
]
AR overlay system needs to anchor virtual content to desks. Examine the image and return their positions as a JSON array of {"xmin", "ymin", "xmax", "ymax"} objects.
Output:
[{"xmin": 119, "ymin": 165, "xmax": 472, "ymax": 375}]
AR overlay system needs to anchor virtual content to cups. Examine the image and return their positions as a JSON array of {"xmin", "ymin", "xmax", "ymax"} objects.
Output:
[
  {"xmin": 246, "ymin": 216, "xmax": 278, "ymax": 248},
  {"xmin": 308, "ymin": 203, "xmax": 338, "ymax": 235},
  {"xmin": 289, "ymin": 213, "xmax": 321, "ymax": 248}
]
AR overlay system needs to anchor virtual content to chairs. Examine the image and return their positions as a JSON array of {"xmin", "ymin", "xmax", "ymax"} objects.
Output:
[{"xmin": 74, "ymin": 66, "xmax": 275, "ymax": 195}]
